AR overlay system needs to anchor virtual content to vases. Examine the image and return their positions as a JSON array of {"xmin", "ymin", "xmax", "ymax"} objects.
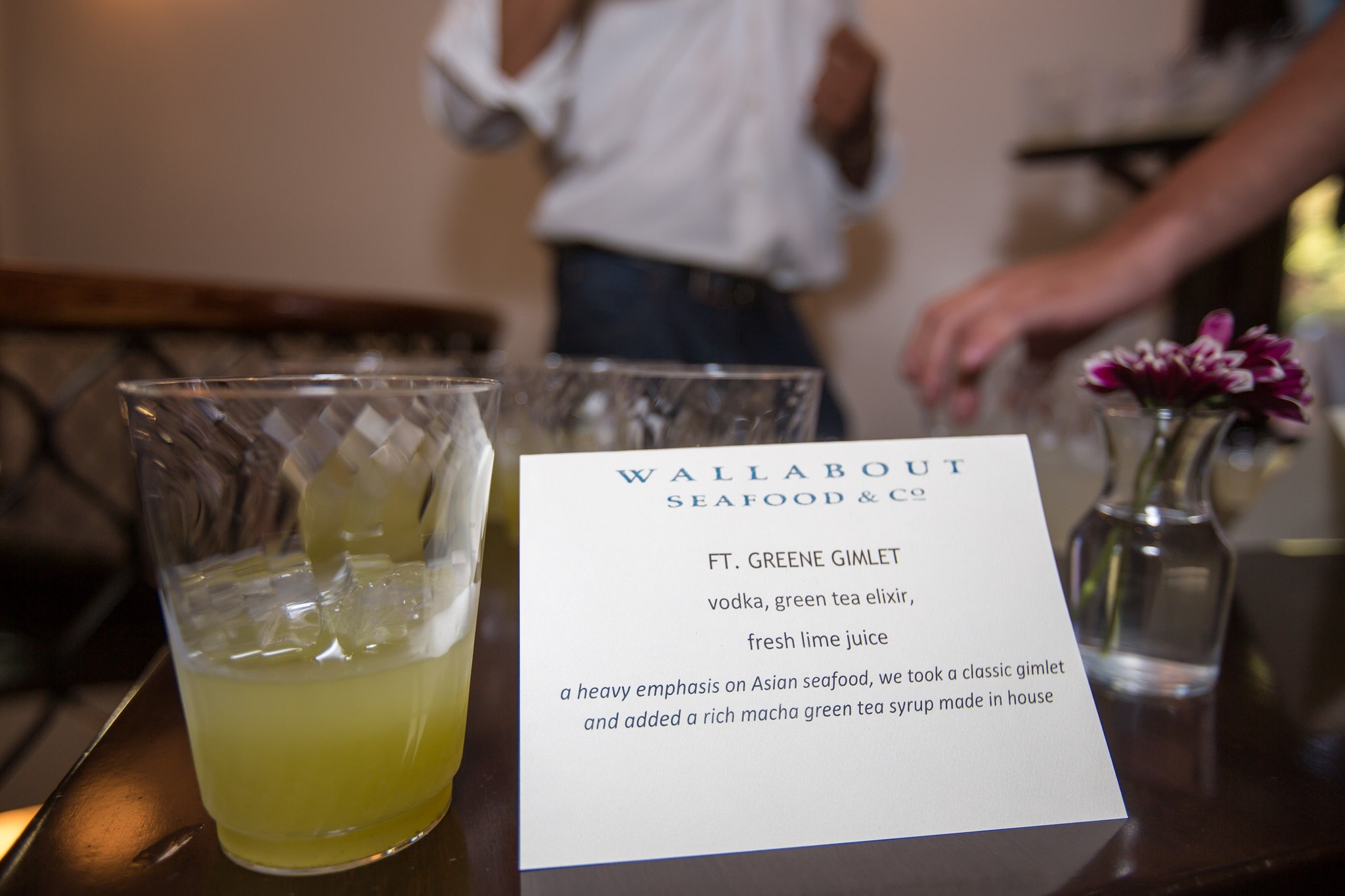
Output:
[{"xmin": 1058, "ymin": 406, "xmax": 1238, "ymax": 694}]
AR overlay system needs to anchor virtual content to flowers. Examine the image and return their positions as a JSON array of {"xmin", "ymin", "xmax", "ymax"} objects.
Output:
[{"xmin": 1072, "ymin": 302, "xmax": 1316, "ymax": 658}]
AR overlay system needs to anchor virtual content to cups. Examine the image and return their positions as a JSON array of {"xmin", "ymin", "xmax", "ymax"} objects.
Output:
[
  {"xmin": 268, "ymin": 351, "xmax": 823, "ymax": 552},
  {"xmin": 115, "ymin": 372, "xmax": 503, "ymax": 877}
]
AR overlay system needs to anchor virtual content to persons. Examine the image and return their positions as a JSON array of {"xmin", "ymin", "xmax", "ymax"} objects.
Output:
[
  {"xmin": 904, "ymin": 0, "xmax": 1345, "ymax": 426},
  {"xmin": 416, "ymin": 0, "xmax": 887, "ymax": 440}
]
